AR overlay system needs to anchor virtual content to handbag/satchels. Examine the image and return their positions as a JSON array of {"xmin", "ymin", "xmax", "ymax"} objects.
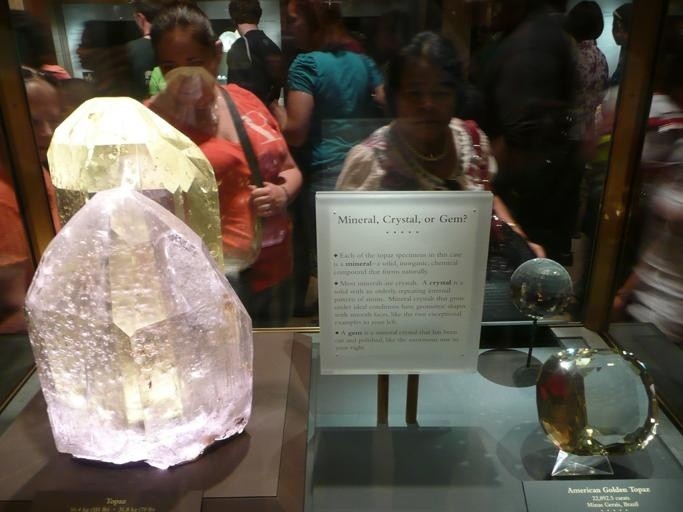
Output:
[
  {"xmin": 483, "ymin": 213, "xmax": 536, "ymax": 321},
  {"xmin": 246, "ymin": 210, "xmax": 294, "ymax": 294}
]
